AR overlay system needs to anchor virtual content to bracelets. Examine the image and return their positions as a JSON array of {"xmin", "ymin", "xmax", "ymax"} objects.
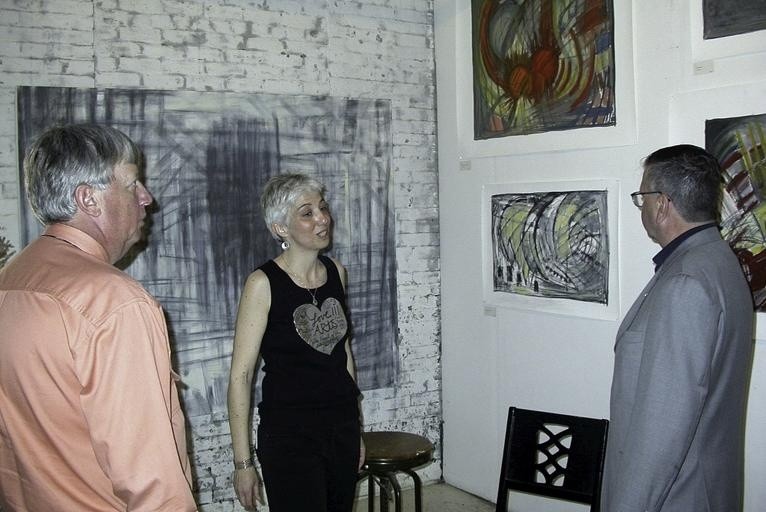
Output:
[{"xmin": 226, "ymin": 461, "xmax": 256, "ymax": 471}]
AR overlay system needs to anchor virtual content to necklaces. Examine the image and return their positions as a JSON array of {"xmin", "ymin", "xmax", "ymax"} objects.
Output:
[{"xmin": 279, "ymin": 255, "xmax": 326, "ymax": 308}]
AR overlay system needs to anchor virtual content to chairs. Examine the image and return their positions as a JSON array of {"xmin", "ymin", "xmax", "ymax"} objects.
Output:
[{"xmin": 495, "ymin": 407, "xmax": 609, "ymax": 512}]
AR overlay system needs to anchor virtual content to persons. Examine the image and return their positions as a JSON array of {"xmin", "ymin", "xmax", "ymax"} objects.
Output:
[
  {"xmin": 225, "ymin": 174, "xmax": 365, "ymax": 511},
  {"xmin": 594, "ymin": 145, "xmax": 759, "ymax": 510},
  {"xmin": 2, "ymin": 123, "xmax": 205, "ymax": 511}
]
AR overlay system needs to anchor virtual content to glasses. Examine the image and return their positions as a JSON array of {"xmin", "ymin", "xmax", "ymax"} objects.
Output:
[{"xmin": 629, "ymin": 191, "xmax": 672, "ymax": 208}]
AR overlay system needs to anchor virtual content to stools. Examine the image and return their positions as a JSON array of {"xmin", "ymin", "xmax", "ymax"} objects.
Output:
[{"xmin": 356, "ymin": 432, "xmax": 432, "ymax": 512}]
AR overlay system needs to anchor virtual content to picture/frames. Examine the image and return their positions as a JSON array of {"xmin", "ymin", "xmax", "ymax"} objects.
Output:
[
  {"xmin": 482, "ymin": 179, "xmax": 622, "ymax": 322},
  {"xmin": 667, "ymin": 82, "xmax": 766, "ymax": 343},
  {"xmin": 455, "ymin": 0, "xmax": 639, "ymax": 160},
  {"xmin": 691, "ymin": 0, "xmax": 766, "ymax": 63}
]
